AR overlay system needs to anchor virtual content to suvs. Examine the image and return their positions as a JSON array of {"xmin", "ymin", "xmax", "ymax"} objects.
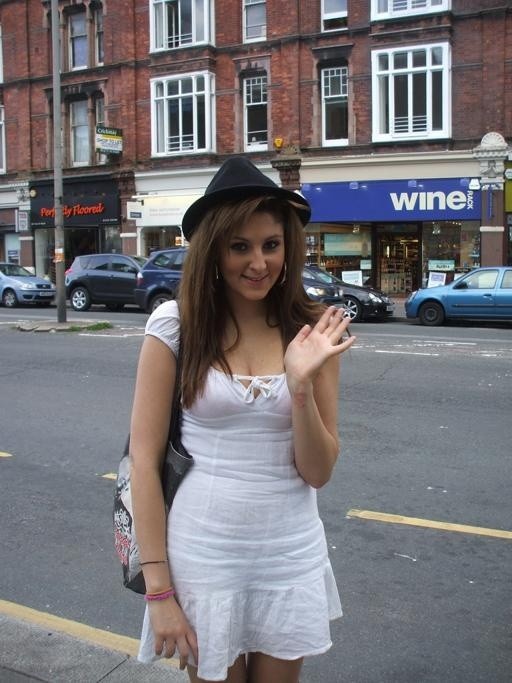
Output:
[{"xmin": 63, "ymin": 250, "xmax": 150, "ymax": 313}]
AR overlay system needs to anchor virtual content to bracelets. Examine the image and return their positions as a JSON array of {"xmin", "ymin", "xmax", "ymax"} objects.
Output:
[
  {"xmin": 139, "ymin": 560, "xmax": 170, "ymax": 567},
  {"xmin": 144, "ymin": 587, "xmax": 175, "ymax": 601}
]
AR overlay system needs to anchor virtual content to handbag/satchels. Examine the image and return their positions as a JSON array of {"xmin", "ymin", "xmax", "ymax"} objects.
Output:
[{"xmin": 113, "ymin": 419, "xmax": 194, "ymax": 595}]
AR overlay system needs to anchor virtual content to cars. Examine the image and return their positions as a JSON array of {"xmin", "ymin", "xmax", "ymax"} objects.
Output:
[
  {"xmin": 301, "ymin": 261, "xmax": 398, "ymax": 323},
  {"xmin": 401, "ymin": 262, "xmax": 512, "ymax": 326},
  {"xmin": 0, "ymin": 260, "xmax": 57, "ymax": 308}
]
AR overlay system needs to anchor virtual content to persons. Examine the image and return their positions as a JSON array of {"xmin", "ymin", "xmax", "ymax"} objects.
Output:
[{"xmin": 113, "ymin": 155, "xmax": 356, "ymax": 683}]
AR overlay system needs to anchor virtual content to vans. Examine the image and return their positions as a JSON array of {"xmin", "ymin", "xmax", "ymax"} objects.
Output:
[{"xmin": 135, "ymin": 246, "xmax": 345, "ymax": 325}]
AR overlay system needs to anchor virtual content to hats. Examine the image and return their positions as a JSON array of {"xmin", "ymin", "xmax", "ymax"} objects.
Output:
[{"xmin": 181, "ymin": 156, "xmax": 311, "ymax": 243}]
